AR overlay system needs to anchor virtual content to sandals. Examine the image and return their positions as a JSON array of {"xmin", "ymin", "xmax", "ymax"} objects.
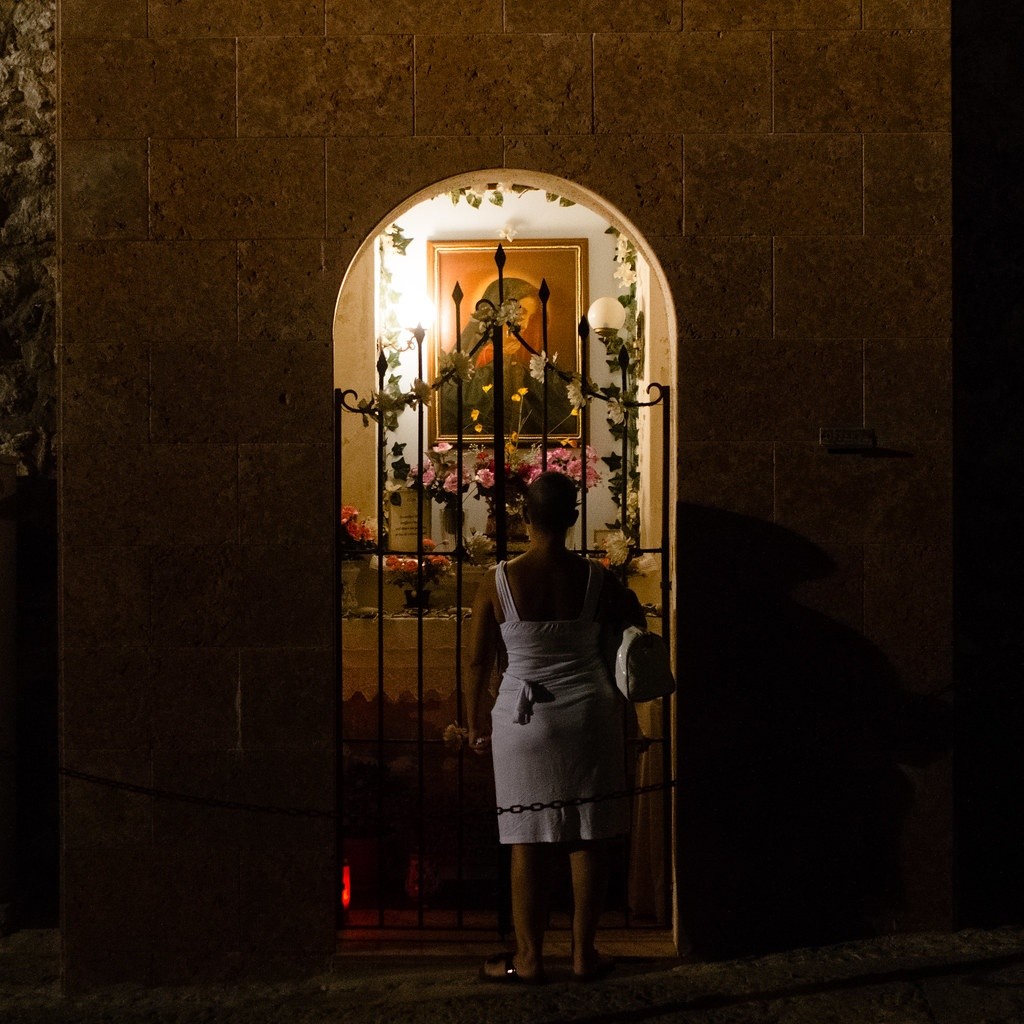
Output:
[
  {"xmin": 568, "ymin": 943, "xmax": 613, "ymax": 982},
  {"xmin": 477, "ymin": 957, "xmax": 549, "ymax": 988}
]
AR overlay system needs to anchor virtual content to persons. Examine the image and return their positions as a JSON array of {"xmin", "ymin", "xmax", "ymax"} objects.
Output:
[{"xmin": 463, "ymin": 470, "xmax": 647, "ymax": 986}]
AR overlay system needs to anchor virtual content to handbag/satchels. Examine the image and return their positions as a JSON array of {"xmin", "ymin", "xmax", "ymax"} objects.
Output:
[{"xmin": 600, "ymin": 619, "xmax": 676, "ymax": 704}]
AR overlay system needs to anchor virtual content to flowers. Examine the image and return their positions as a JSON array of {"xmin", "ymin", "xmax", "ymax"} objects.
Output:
[
  {"xmin": 338, "ymin": 179, "xmax": 658, "ymax": 591},
  {"xmin": 443, "ymin": 723, "xmax": 472, "ymax": 747}
]
